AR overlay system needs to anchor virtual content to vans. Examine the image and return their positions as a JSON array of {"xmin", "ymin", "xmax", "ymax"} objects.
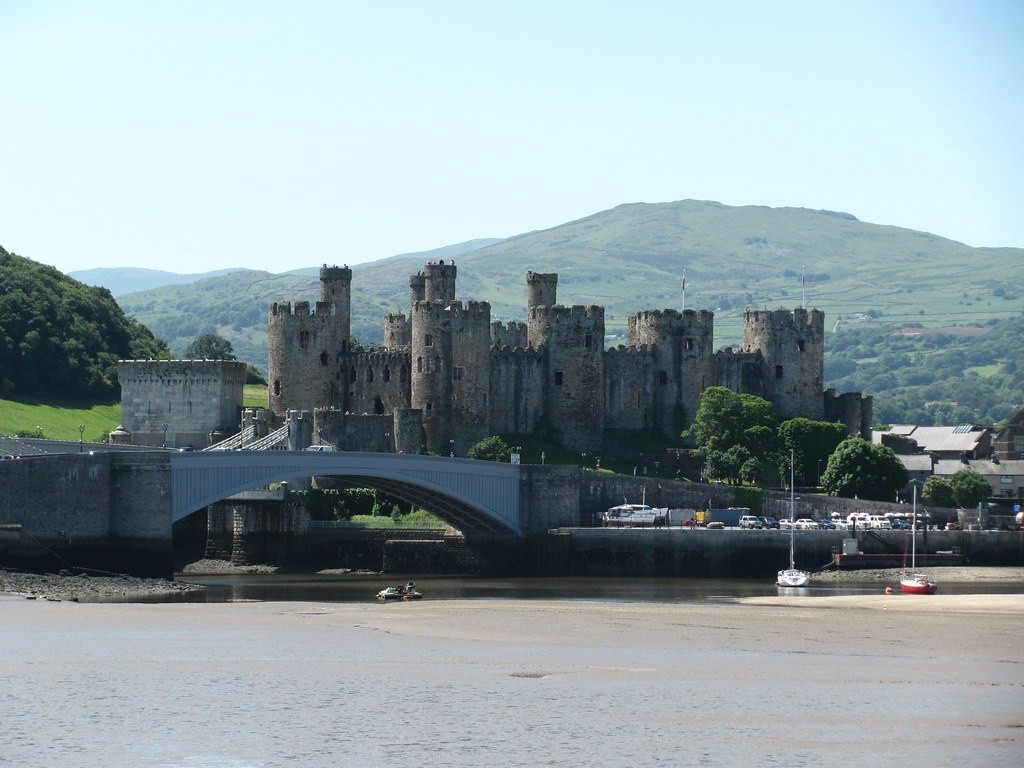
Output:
[
  {"xmin": 846, "ymin": 514, "xmax": 871, "ymax": 530},
  {"xmin": 865, "ymin": 515, "xmax": 893, "ymax": 532}
]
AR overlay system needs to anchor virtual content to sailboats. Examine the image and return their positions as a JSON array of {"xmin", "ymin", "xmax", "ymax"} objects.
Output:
[
  {"xmin": 898, "ymin": 485, "xmax": 938, "ymax": 594},
  {"xmin": 777, "ymin": 448, "xmax": 811, "ymax": 587}
]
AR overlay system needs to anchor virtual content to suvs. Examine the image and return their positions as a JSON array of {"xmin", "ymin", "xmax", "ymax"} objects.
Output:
[{"xmin": 739, "ymin": 516, "xmax": 763, "ymax": 529}]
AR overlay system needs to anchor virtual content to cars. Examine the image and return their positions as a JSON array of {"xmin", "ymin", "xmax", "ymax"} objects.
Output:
[
  {"xmin": 831, "ymin": 519, "xmax": 850, "ymax": 530},
  {"xmin": 758, "ymin": 516, "xmax": 780, "ymax": 529},
  {"xmin": 816, "ymin": 519, "xmax": 837, "ymax": 530},
  {"xmin": 887, "ymin": 516, "xmax": 911, "ymax": 531},
  {"xmin": 778, "ymin": 518, "xmax": 801, "ymax": 530},
  {"xmin": 795, "ymin": 518, "xmax": 819, "ymax": 532}
]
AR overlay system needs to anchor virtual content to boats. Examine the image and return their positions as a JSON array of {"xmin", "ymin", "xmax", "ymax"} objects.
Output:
[{"xmin": 376, "ymin": 580, "xmax": 423, "ymax": 602}]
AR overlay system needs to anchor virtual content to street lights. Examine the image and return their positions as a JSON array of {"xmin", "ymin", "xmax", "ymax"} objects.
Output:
[
  {"xmin": 385, "ymin": 431, "xmax": 391, "ymax": 454},
  {"xmin": 317, "ymin": 427, "xmax": 323, "ymax": 446},
  {"xmin": 596, "ymin": 456, "xmax": 602, "ymax": 471},
  {"xmin": 450, "ymin": 438, "xmax": 455, "ymax": 458},
  {"xmin": 517, "ymin": 445, "xmax": 522, "ymax": 455},
  {"xmin": 78, "ymin": 425, "xmax": 85, "ymax": 453},
  {"xmin": 161, "ymin": 422, "xmax": 168, "ymax": 451},
  {"xmin": 581, "ymin": 452, "xmax": 587, "ymax": 470}
]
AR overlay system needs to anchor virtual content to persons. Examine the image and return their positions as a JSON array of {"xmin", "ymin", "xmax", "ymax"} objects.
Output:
[{"xmin": 690, "ymin": 516, "xmax": 697, "ymax": 530}]
